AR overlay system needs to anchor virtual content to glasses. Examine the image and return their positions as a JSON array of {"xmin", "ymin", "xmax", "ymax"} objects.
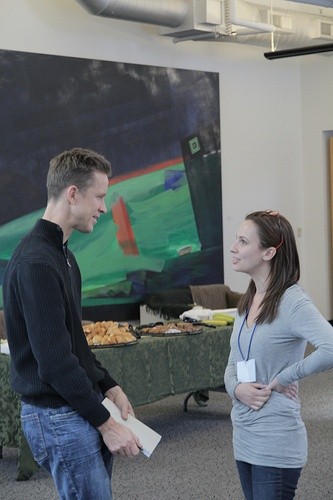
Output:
[{"xmin": 265, "ymin": 209, "xmax": 283, "ymax": 249}]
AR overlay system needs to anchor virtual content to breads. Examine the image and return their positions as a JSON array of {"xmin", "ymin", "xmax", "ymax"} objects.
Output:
[{"xmin": 83, "ymin": 320, "xmax": 137, "ymax": 346}]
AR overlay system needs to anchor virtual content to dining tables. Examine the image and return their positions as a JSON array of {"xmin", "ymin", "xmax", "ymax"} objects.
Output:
[{"xmin": 0, "ymin": 326, "xmax": 233, "ymax": 481}]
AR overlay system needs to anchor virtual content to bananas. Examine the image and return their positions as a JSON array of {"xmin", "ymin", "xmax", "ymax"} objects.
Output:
[{"xmin": 201, "ymin": 313, "xmax": 234, "ymax": 326}]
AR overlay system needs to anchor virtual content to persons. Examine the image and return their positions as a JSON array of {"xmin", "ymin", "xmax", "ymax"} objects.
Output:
[
  {"xmin": 222, "ymin": 209, "xmax": 333, "ymax": 500},
  {"xmin": 1, "ymin": 148, "xmax": 143, "ymax": 500}
]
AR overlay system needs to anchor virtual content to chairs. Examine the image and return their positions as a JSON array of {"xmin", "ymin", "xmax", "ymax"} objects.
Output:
[
  {"xmin": 189, "ymin": 284, "xmax": 242, "ymax": 310},
  {"xmin": 145, "ymin": 291, "xmax": 194, "ymax": 318}
]
